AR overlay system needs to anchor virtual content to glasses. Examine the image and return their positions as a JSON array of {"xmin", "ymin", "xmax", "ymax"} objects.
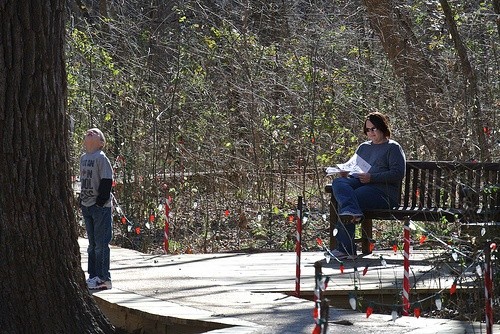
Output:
[{"xmin": 364, "ymin": 127, "xmax": 379, "ymax": 132}]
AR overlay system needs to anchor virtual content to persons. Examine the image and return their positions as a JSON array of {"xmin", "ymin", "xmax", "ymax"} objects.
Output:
[
  {"xmin": 79, "ymin": 128, "xmax": 115, "ymax": 290},
  {"xmin": 324, "ymin": 112, "xmax": 407, "ymax": 259}
]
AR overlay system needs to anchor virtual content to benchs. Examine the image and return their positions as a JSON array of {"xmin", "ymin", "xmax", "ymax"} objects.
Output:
[{"xmin": 325, "ymin": 160, "xmax": 500, "ymax": 260}]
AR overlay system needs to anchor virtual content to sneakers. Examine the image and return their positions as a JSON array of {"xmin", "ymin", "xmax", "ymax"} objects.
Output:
[
  {"xmin": 324, "ymin": 249, "xmax": 359, "ymax": 260},
  {"xmin": 85, "ymin": 276, "xmax": 112, "ymax": 290}
]
